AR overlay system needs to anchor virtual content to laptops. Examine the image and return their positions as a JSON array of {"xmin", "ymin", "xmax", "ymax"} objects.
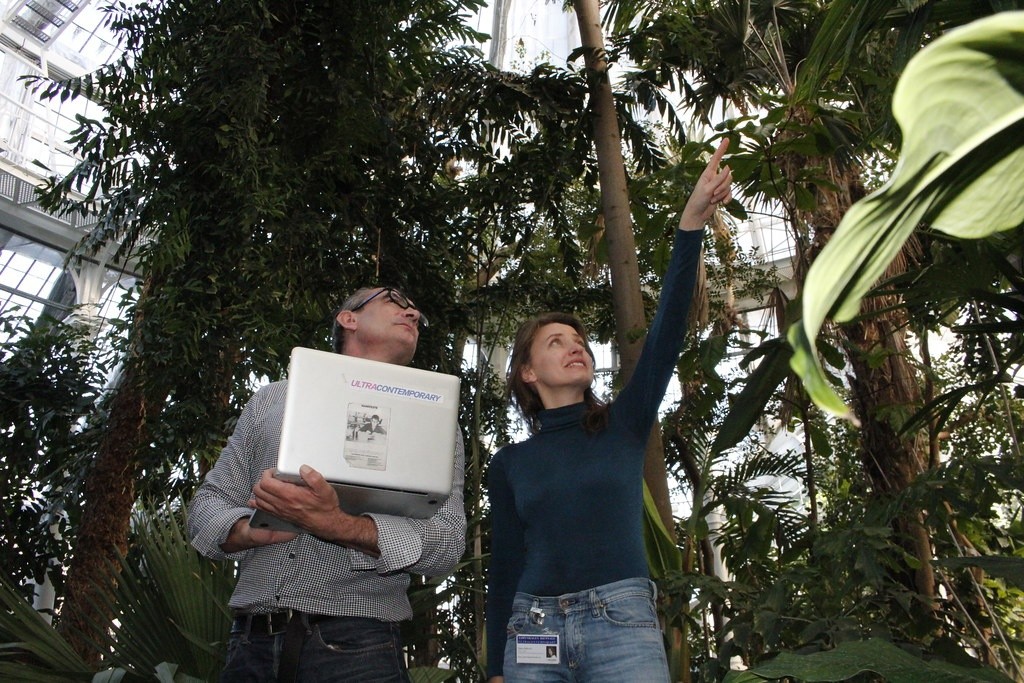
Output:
[{"xmin": 247, "ymin": 347, "xmax": 460, "ymax": 532}]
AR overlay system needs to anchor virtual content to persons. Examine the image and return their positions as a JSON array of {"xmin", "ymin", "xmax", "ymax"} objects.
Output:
[
  {"xmin": 184, "ymin": 286, "xmax": 469, "ymax": 683},
  {"xmin": 484, "ymin": 138, "xmax": 734, "ymax": 683}
]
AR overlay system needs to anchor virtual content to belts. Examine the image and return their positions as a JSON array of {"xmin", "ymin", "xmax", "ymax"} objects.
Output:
[{"xmin": 229, "ymin": 610, "xmax": 335, "ymax": 639}]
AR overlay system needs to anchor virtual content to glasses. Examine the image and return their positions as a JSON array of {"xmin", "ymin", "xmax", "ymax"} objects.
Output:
[{"xmin": 350, "ymin": 287, "xmax": 429, "ymax": 327}]
